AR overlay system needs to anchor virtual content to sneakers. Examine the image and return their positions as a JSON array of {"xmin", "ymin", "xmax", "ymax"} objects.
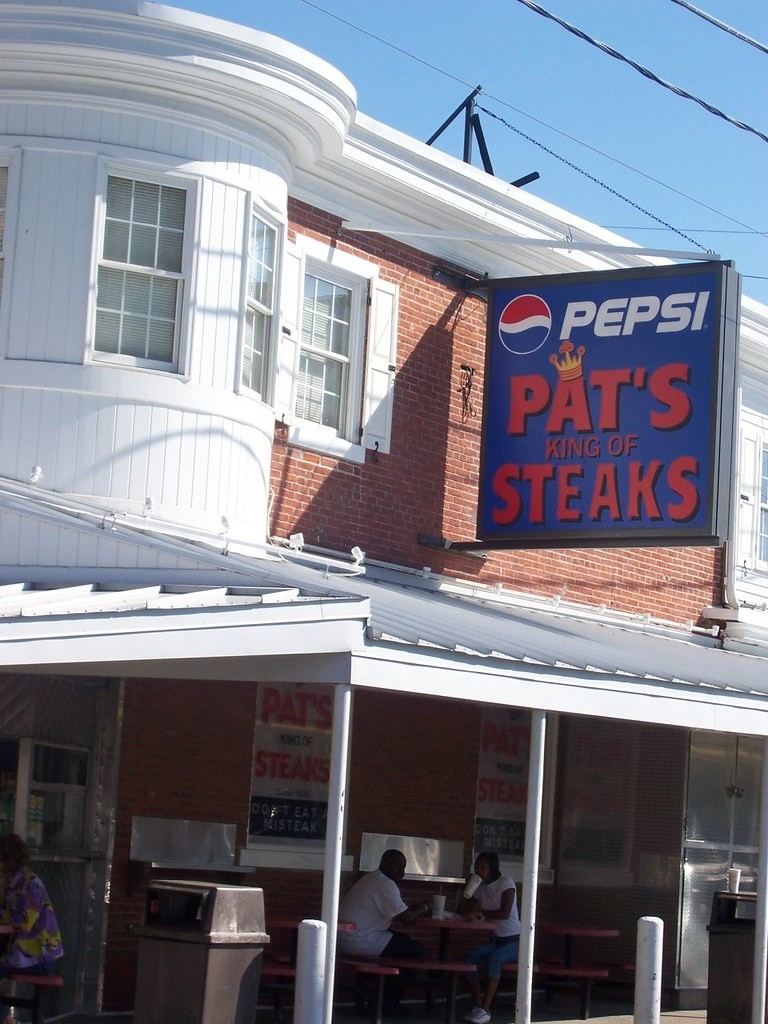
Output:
[{"xmin": 463, "ymin": 1007, "xmax": 491, "ymax": 1024}]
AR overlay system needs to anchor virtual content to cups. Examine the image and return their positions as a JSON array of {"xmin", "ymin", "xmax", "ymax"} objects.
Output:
[
  {"xmin": 462, "ymin": 874, "xmax": 482, "ymax": 899},
  {"xmin": 728, "ymin": 868, "xmax": 741, "ymax": 892},
  {"xmin": 432, "ymin": 895, "xmax": 446, "ymax": 919}
]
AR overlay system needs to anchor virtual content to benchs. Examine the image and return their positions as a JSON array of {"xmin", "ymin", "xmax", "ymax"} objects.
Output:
[
  {"xmin": 259, "ymin": 953, "xmax": 635, "ymax": 1024},
  {"xmin": 0, "ymin": 972, "xmax": 63, "ymax": 1024}
]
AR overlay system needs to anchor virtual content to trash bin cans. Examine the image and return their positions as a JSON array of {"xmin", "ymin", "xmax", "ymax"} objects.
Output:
[
  {"xmin": 707, "ymin": 892, "xmax": 768, "ymax": 1024},
  {"xmin": 132, "ymin": 878, "xmax": 271, "ymax": 1024}
]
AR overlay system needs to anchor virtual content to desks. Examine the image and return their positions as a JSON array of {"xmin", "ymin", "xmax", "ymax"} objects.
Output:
[
  {"xmin": 393, "ymin": 916, "xmax": 504, "ymax": 964},
  {"xmin": 536, "ymin": 922, "xmax": 619, "ymax": 970},
  {"xmin": 0, "ymin": 923, "xmax": 16, "ymax": 934},
  {"xmin": 263, "ymin": 917, "xmax": 356, "ymax": 982}
]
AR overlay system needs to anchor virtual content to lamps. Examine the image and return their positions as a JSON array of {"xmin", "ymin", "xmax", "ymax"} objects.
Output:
[
  {"xmin": 25, "ymin": 466, "xmax": 44, "ymax": 486},
  {"xmin": 219, "ymin": 516, "xmax": 230, "ymax": 536},
  {"xmin": 349, "ymin": 545, "xmax": 365, "ymax": 568},
  {"xmin": 142, "ymin": 497, "xmax": 155, "ymax": 518},
  {"xmin": 289, "ymin": 533, "xmax": 307, "ymax": 552}
]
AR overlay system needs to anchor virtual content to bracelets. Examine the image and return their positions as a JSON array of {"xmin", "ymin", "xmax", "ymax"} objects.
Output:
[
  {"xmin": 482, "ymin": 911, "xmax": 485, "ymax": 916},
  {"xmin": 421, "ymin": 903, "xmax": 428, "ymax": 910}
]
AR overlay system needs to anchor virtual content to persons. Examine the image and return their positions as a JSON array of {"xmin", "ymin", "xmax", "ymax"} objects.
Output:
[
  {"xmin": 556, "ymin": 839, "xmax": 612, "ymax": 895},
  {"xmin": 0, "ymin": 833, "xmax": 64, "ymax": 1024},
  {"xmin": 37, "ymin": 744, "xmax": 88, "ymax": 849},
  {"xmin": 438, "ymin": 851, "xmax": 521, "ymax": 1024},
  {"xmin": 336, "ymin": 849, "xmax": 434, "ymax": 1018}
]
